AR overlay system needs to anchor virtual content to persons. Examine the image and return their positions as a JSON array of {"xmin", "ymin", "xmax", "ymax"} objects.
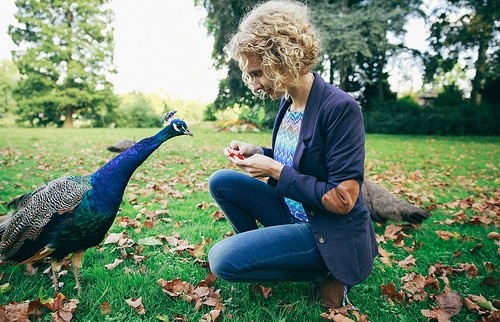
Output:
[{"xmin": 207, "ymin": 0, "xmax": 378, "ymax": 309}]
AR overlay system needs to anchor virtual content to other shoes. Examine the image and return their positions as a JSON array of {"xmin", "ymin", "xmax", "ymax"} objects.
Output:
[{"xmin": 320, "ymin": 274, "xmax": 351, "ymax": 309}]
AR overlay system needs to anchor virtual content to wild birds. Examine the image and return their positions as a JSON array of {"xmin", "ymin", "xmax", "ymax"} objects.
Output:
[{"xmin": 0, "ymin": 110, "xmax": 193, "ymax": 295}]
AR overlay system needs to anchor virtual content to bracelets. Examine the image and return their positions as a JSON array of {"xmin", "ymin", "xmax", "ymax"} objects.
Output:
[{"xmin": 258, "ymin": 147, "xmax": 264, "ymax": 156}]
